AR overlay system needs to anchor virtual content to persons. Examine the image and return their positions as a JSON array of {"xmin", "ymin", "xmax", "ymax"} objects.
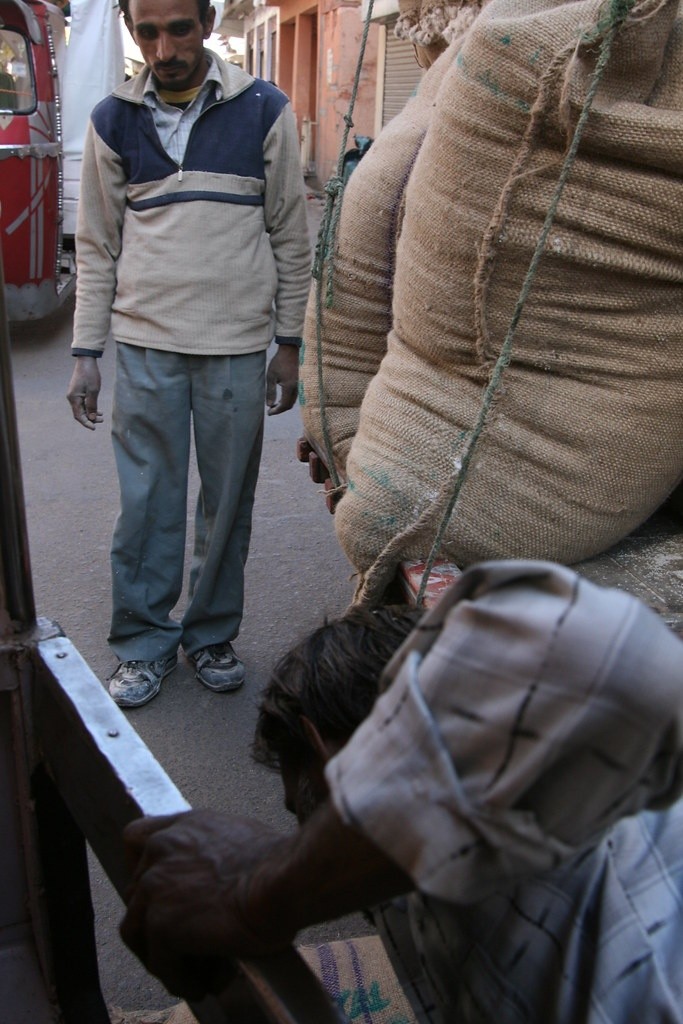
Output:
[
  {"xmin": 67, "ymin": 0, "xmax": 309, "ymax": 708},
  {"xmin": 115, "ymin": 560, "xmax": 683, "ymax": 1023}
]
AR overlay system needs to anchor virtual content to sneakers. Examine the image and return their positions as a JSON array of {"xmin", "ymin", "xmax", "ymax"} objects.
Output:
[
  {"xmin": 106, "ymin": 652, "xmax": 178, "ymax": 707},
  {"xmin": 188, "ymin": 640, "xmax": 246, "ymax": 691}
]
